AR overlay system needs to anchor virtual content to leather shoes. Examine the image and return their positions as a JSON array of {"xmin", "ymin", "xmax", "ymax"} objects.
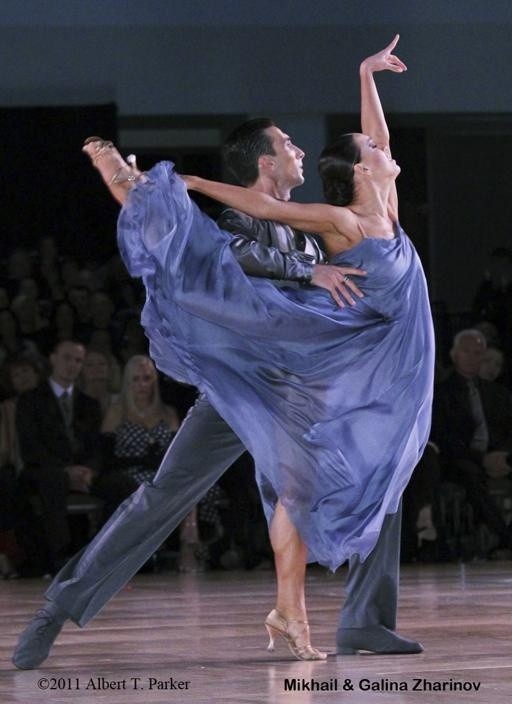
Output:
[{"xmin": 175, "ymin": 537, "xmax": 213, "ymax": 562}]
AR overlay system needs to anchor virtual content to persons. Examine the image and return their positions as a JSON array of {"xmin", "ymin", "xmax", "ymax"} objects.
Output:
[
  {"xmin": 9, "ymin": 116, "xmax": 427, "ymax": 673},
  {"xmin": 76, "ymin": 32, "xmax": 409, "ymax": 663}
]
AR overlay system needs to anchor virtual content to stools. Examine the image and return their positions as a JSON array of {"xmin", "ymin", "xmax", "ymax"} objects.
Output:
[
  {"xmin": 440, "ymin": 479, "xmax": 510, "ymax": 559},
  {"xmin": 25, "ymin": 492, "xmax": 101, "ymax": 563}
]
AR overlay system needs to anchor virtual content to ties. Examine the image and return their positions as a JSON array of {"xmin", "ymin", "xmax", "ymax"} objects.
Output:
[
  {"xmin": 466, "ymin": 381, "xmax": 489, "ymax": 452},
  {"xmin": 58, "ymin": 392, "xmax": 76, "ymax": 449}
]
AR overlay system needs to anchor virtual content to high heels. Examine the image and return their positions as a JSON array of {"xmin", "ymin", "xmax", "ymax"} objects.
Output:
[
  {"xmin": 334, "ymin": 616, "xmax": 424, "ymax": 657},
  {"xmin": 84, "ymin": 137, "xmax": 143, "ymax": 188},
  {"xmin": 265, "ymin": 605, "xmax": 327, "ymax": 662},
  {"xmin": 12, "ymin": 610, "xmax": 66, "ymax": 672}
]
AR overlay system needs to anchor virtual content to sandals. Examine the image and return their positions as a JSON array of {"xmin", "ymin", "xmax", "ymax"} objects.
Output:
[{"xmin": 0, "ymin": 570, "xmax": 25, "ymax": 582}]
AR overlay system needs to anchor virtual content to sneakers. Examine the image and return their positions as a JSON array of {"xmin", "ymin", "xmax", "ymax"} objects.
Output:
[{"xmin": 417, "ymin": 525, "xmax": 437, "ymax": 549}]
